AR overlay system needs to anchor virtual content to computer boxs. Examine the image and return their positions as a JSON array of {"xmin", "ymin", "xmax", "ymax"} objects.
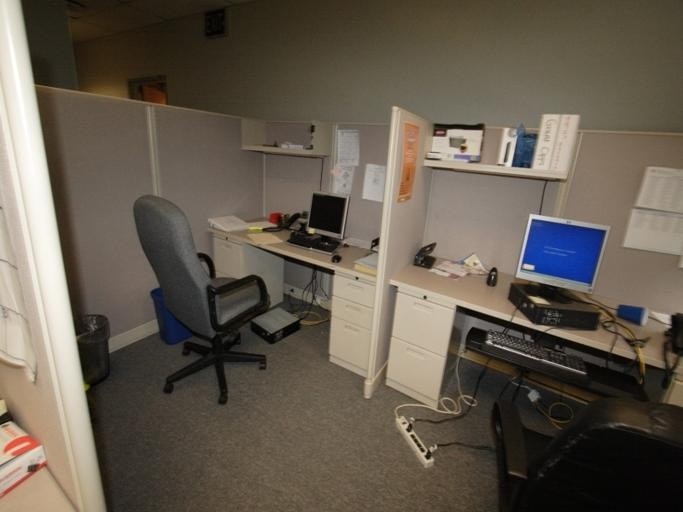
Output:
[
  {"xmin": 507, "ymin": 282, "xmax": 602, "ymax": 330},
  {"xmin": 250, "ymin": 307, "xmax": 301, "ymax": 344}
]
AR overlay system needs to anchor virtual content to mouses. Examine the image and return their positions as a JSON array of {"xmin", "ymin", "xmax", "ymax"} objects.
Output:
[{"xmin": 332, "ymin": 255, "xmax": 341, "ymax": 263}]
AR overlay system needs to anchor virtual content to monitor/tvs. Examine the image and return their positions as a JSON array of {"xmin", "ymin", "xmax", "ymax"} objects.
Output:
[
  {"xmin": 514, "ymin": 213, "xmax": 611, "ymax": 303},
  {"xmin": 305, "ymin": 190, "xmax": 350, "ymax": 247}
]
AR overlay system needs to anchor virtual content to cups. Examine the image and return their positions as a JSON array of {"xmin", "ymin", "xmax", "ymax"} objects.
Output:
[{"xmin": 615, "ymin": 302, "xmax": 649, "ymax": 327}]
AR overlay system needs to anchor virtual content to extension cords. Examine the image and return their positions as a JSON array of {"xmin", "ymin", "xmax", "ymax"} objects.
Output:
[{"xmin": 395, "ymin": 415, "xmax": 434, "ymax": 469}]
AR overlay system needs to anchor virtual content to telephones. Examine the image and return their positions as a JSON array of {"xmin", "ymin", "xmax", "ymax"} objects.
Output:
[{"xmin": 207, "ymin": 215, "xmax": 248, "ymax": 233}]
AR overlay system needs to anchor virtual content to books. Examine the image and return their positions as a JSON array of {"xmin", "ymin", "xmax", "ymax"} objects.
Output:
[
  {"xmin": 425, "ymin": 156, "xmax": 480, "ymax": 164},
  {"xmin": 425, "ymin": 152, "xmax": 482, "ymax": 162},
  {"xmin": 352, "ymin": 252, "xmax": 378, "ymax": 277},
  {"xmin": 427, "ymin": 251, "xmax": 498, "ymax": 282}
]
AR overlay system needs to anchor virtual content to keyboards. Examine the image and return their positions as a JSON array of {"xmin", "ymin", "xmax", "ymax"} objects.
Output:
[{"xmin": 487, "ymin": 330, "xmax": 588, "ymax": 377}]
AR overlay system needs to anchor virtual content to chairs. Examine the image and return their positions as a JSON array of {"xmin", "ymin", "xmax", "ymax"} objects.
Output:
[
  {"xmin": 489, "ymin": 396, "xmax": 682, "ymax": 511},
  {"xmin": 131, "ymin": 194, "xmax": 271, "ymax": 405}
]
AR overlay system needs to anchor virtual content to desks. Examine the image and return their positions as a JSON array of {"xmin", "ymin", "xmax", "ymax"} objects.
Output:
[
  {"xmin": 385, "ymin": 253, "xmax": 683, "ymax": 410},
  {"xmin": 205, "ymin": 218, "xmax": 378, "ymax": 379}
]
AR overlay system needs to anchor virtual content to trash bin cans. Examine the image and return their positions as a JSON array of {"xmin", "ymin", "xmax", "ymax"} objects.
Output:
[
  {"xmin": 77, "ymin": 314, "xmax": 110, "ymax": 385},
  {"xmin": 149, "ymin": 288, "xmax": 195, "ymax": 344}
]
